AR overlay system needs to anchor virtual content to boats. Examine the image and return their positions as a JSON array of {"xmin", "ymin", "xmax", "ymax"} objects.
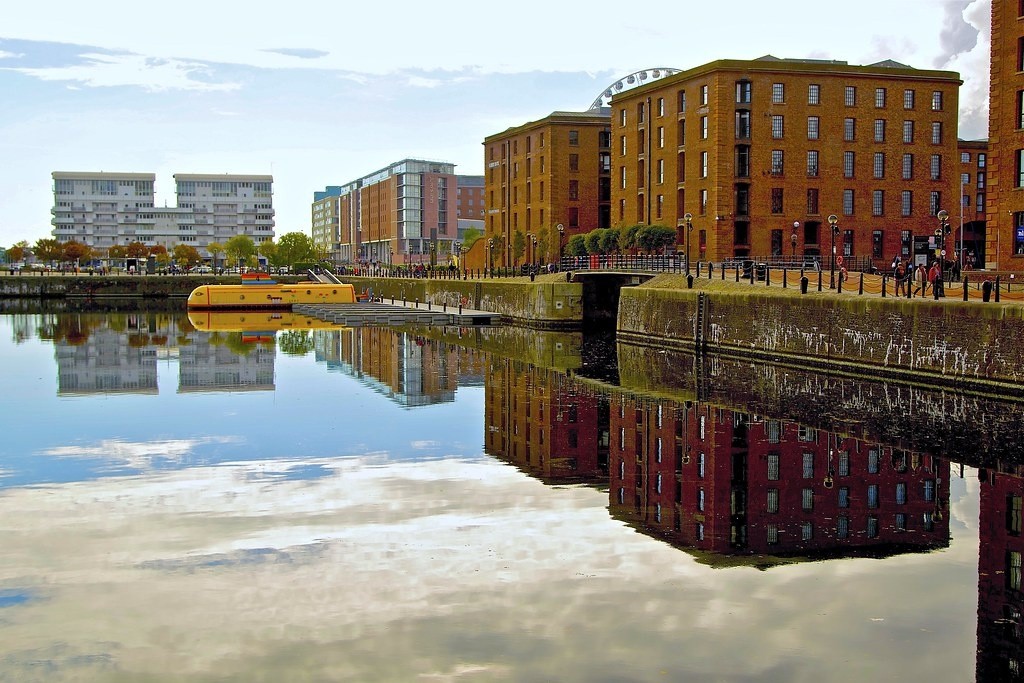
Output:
[{"xmin": 187, "ymin": 272, "xmax": 357, "ymax": 307}]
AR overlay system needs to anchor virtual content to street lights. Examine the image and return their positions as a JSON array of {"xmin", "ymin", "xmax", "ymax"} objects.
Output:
[
  {"xmin": 792, "ymin": 234, "xmax": 797, "ymax": 255},
  {"xmin": 488, "ymin": 238, "xmax": 494, "ymax": 278},
  {"xmin": 456, "ymin": 242, "xmax": 460, "ymax": 277},
  {"xmin": 935, "ymin": 211, "xmax": 952, "ymax": 297},
  {"xmin": 463, "ymin": 246, "xmax": 466, "ymax": 270},
  {"xmin": 828, "ymin": 214, "xmax": 838, "ymax": 289},
  {"xmin": 532, "ymin": 234, "xmax": 537, "ymax": 266},
  {"xmin": 935, "ymin": 229, "xmax": 942, "ymax": 267},
  {"xmin": 485, "ymin": 245, "xmax": 488, "ymax": 278},
  {"xmin": 430, "ymin": 242, "xmax": 434, "ymax": 276},
  {"xmin": 556, "ymin": 224, "xmax": 564, "ymax": 272},
  {"xmin": 685, "ymin": 214, "xmax": 692, "ymax": 276},
  {"xmin": 408, "ymin": 243, "xmax": 413, "ymax": 277}
]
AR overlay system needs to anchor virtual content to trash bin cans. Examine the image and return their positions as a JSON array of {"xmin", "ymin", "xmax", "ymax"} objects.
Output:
[
  {"xmin": 10, "ymin": 269, "xmax": 15, "ymax": 276},
  {"xmin": 755, "ymin": 263, "xmax": 766, "ymax": 281},
  {"xmin": 742, "ymin": 260, "xmax": 753, "ymax": 279},
  {"xmin": 89, "ymin": 269, "xmax": 93, "ymax": 276}
]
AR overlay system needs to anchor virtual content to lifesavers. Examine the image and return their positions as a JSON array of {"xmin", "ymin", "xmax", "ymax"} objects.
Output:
[
  {"xmin": 840, "ymin": 267, "xmax": 848, "ymax": 283},
  {"xmin": 836, "ymin": 256, "xmax": 844, "ymax": 266},
  {"xmin": 462, "ymin": 296, "xmax": 468, "ymax": 305},
  {"xmin": 466, "ymin": 268, "xmax": 470, "ymax": 275}
]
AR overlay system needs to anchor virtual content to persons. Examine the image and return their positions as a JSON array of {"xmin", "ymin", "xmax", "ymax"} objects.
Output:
[
  {"xmin": 941, "ymin": 256, "xmax": 973, "ymax": 282},
  {"xmin": 522, "ymin": 261, "xmax": 555, "ymax": 276},
  {"xmin": 448, "ymin": 263, "xmax": 470, "ymax": 278},
  {"xmin": 130, "ymin": 269, "xmax": 133, "ymax": 275},
  {"xmin": 891, "ymin": 253, "xmax": 942, "ymax": 296},
  {"xmin": 396, "ymin": 262, "xmax": 431, "ymax": 278},
  {"xmin": 56, "ymin": 263, "xmax": 111, "ymax": 275},
  {"xmin": 163, "ymin": 265, "xmax": 187, "ymax": 276},
  {"xmin": 836, "ymin": 256, "xmax": 847, "ymax": 283},
  {"xmin": 336, "ymin": 266, "xmax": 368, "ymax": 276}
]
